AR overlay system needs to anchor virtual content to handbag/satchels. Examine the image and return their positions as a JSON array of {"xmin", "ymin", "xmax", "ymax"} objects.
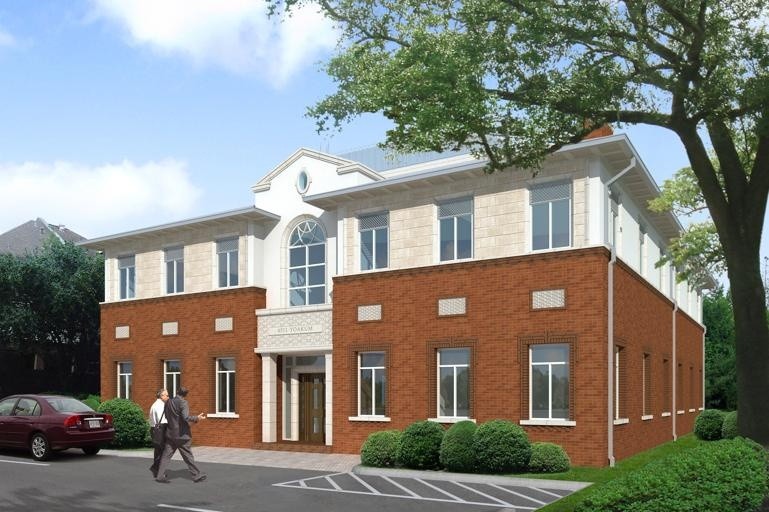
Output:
[{"xmin": 153, "ymin": 424, "xmax": 169, "ymax": 444}]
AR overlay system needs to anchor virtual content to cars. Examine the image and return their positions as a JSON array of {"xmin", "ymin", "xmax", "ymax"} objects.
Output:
[{"xmin": 0, "ymin": 393, "xmax": 116, "ymax": 461}]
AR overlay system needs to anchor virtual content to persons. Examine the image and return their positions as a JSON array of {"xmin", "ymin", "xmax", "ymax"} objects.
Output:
[
  {"xmin": 148, "ymin": 389, "xmax": 170, "ymax": 478},
  {"xmin": 155, "ymin": 386, "xmax": 207, "ymax": 484}
]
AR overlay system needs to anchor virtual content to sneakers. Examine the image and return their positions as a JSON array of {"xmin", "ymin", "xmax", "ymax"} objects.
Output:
[
  {"xmin": 192, "ymin": 473, "xmax": 208, "ymax": 481},
  {"xmin": 149, "ymin": 465, "xmax": 169, "ymax": 484}
]
